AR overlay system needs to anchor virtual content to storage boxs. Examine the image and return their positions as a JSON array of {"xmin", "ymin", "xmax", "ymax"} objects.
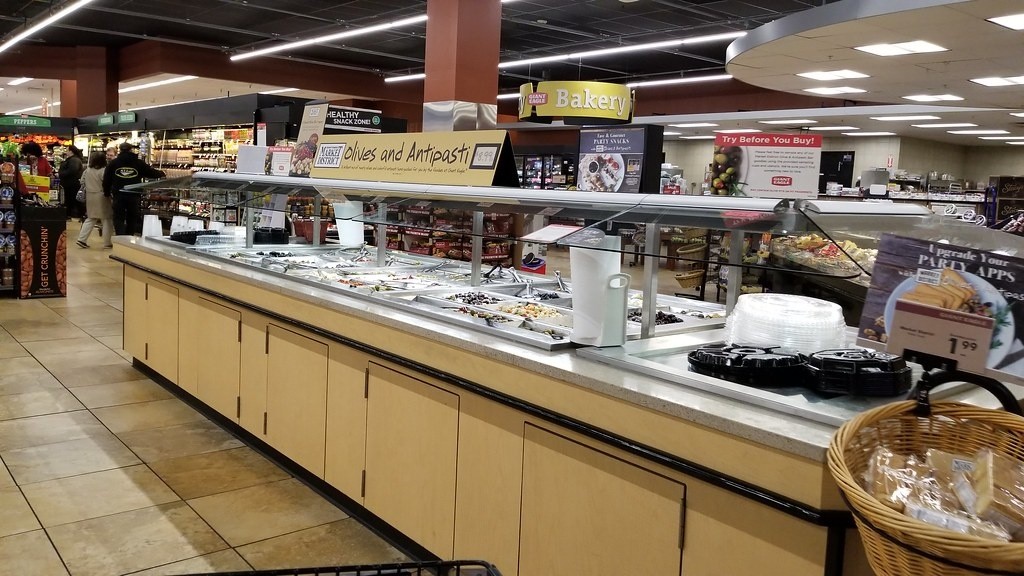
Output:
[{"xmin": 22, "ymin": 175, "xmax": 50, "ymax": 204}]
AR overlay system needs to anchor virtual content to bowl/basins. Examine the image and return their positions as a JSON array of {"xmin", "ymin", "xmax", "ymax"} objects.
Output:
[
  {"xmin": 302, "ymin": 221, "xmax": 328, "ymax": 243},
  {"xmin": 293, "ymin": 221, "xmax": 303, "ymax": 237},
  {"xmin": 142, "ymin": 214, "xmax": 224, "ymax": 238},
  {"xmin": 588, "ymin": 161, "xmax": 601, "ymax": 174}
]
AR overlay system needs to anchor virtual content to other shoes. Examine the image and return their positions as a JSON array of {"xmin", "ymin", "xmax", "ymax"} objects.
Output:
[
  {"xmin": 76, "ymin": 241, "xmax": 90, "ymax": 248},
  {"xmin": 103, "ymin": 244, "xmax": 113, "ymax": 248}
]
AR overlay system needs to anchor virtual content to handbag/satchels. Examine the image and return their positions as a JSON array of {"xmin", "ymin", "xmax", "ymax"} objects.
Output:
[{"xmin": 76, "ymin": 170, "xmax": 87, "ymax": 203}]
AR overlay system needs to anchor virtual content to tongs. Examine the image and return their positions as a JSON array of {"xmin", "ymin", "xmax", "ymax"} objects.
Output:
[
  {"xmin": 484, "ymin": 264, "xmax": 502, "ymax": 278},
  {"xmin": 351, "ymin": 250, "xmax": 368, "ymax": 262},
  {"xmin": 424, "ymin": 262, "xmax": 445, "ymax": 273},
  {"xmin": 552, "ymin": 271, "xmax": 571, "ymax": 294},
  {"xmin": 385, "ymin": 258, "xmax": 397, "ymax": 268},
  {"xmin": 508, "ymin": 267, "xmax": 523, "ymax": 283},
  {"xmin": 525, "ymin": 279, "xmax": 533, "ymax": 295}
]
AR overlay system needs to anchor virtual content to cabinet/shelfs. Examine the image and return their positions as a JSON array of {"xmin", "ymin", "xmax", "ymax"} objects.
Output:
[
  {"xmin": 107, "ymin": 243, "xmax": 876, "ymax": 576},
  {"xmin": 385, "ymin": 205, "xmax": 516, "ymax": 268},
  {"xmin": 0, "ymin": 155, "xmax": 18, "ymax": 300}
]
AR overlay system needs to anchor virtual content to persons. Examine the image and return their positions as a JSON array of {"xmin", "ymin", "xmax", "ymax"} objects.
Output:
[
  {"xmin": 21, "ymin": 142, "xmax": 118, "ymax": 249},
  {"xmin": 103, "ymin": 142, "xmax": 167, "ymax": 235}
]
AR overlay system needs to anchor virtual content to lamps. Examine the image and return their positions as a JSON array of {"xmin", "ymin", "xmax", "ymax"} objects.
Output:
[{"xmin": 0, "ymin": 0, "xmax": 751, "ymax": 115}]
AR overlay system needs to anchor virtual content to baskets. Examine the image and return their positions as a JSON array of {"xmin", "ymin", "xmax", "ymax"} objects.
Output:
[{"xmin": 826, "ymin": 370, "xmax": 1024, "ymax": 576}]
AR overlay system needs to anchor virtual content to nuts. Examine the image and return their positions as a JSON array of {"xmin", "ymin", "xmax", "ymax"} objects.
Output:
[{"xmin": 19, "ymin": 230, "xmax": 67, "ymax": 297}]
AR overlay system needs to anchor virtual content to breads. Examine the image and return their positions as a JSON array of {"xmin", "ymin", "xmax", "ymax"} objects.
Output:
[
  {"xmin": 900, "ymin": 267, "xmax": 977, "ymax": 312},
  {"xmin": 797, "ymin": 234, "xmax": 866, "ymax": 266},
  {"xmin": 867, "ymin": 445, "xmax": 1024, "ymax": 547}
]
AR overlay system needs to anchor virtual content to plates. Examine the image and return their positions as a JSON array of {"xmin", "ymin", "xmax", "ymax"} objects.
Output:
[
  {"xmin": 579, "ymin": 153, "xmax": 625, "ymax": 193},
  {"xmin": 522, "ymin": 257, "xmax": 544, "ymax": 267},
  {"xmin": 733, "ymin": 146, "xmax": 749, "ymax": 196},
  {"xmin": 883, "ymin": 268, "xmax": 1015, "ymax": 369}
]
